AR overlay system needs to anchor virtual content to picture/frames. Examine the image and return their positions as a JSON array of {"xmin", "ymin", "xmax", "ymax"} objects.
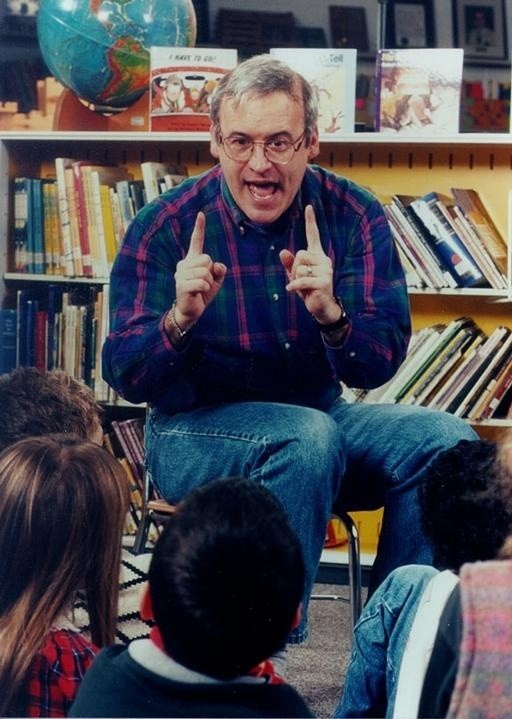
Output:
[{"xmin": 379, "ymin": 3, "xmax": 505, "ymax": 62}]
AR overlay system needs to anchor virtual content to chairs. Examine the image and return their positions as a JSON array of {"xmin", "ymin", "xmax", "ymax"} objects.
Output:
[{"xmin": 130, "ymin": 465, "xmax": 362, "ymax": 634}]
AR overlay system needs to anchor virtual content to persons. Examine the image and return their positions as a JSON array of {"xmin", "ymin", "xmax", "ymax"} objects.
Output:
[{"xmin": 0, "ymin": 53, "xmax": 512, "ymax": 718}]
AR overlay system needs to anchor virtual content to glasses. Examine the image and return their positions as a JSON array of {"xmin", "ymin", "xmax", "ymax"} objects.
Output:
[{"xmin": 221, "ymin": 130, "xmax": 308, "ymax": 165}]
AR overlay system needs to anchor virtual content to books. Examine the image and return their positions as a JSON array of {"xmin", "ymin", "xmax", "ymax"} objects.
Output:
[{"xmin": 0, "ymin": 46, "xmax": 512, "ymax": 544}]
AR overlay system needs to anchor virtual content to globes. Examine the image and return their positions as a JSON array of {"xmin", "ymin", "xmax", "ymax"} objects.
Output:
[{"xmin": 36, "ymin": 0, "xmax": 198, "ymax": 119}]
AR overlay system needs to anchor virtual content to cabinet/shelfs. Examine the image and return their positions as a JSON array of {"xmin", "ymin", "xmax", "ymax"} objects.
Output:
[{"xmin": 3, "ymin": 128, "xmax": 510, "ymax": 587}]
